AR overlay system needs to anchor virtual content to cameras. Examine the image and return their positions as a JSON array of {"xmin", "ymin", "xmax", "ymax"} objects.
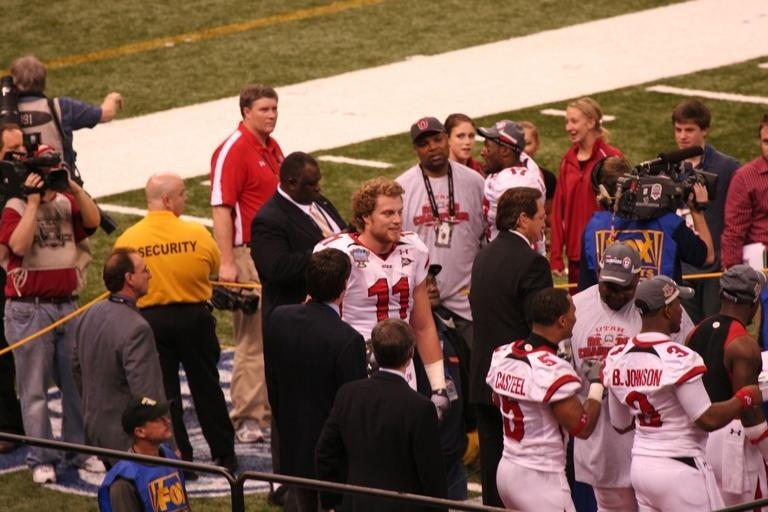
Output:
[{"xmin": 87, "ymin": 199, "xmax": 116, "ymax": 235}]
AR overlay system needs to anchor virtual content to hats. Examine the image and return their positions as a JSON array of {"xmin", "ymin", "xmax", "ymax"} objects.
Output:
[
  {"xmin": 476, "ymin": 119, "xmax": 525, "ymax": 153},
  {"xmin": 410, "ymin": 118, "xmax": 448, "ymax": 144},
  {"xmin": 634, "ymin": 275, "xmax": 696, "ymax": 316},
  {"xmin": 719, "ymin": 264, "xmax": 767, "ymax": 305},
  {"xmin": 121, "ymin": 396, "xmax": 175, "ymax": 434},
  {"xmin": 598, "ymin": 242, "xmax": 642, "ymax": 287},
  {"xmin": 429, "ymin": 264, "xmax": 442, "ymax": 276}
]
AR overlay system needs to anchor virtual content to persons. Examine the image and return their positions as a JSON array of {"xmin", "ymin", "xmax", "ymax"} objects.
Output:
[
  {"xmin": 603, "ymin": 273, "xmax": 763, "ymax": 511},
  {"xmin": 315, "ymin": 317, "xmax": 450, "ymax": 512},
  {"xmin": 483, "ymin": 288, "xmax": 604, "ymax": 512},
  {"xmin": 262, "ymin": 251, "xmax": 370, "ymax": 510},
  {"xmin": 98, "ymin": 396, "xmax": 189, "ymax": 512},
  {"xmin": 683, "ymin": 265, "xmax": 767, "ymax": 512},
  {"xmin": 553, "ymin": 243, "xmax": 696, "ymax": 512},
  {"xmin": 1, "ymin": 54, "xmax": 767, "ymax": 488},
  {"xmin": 466, "ymin": 185, "xmax": 553, "ymax": 512}
]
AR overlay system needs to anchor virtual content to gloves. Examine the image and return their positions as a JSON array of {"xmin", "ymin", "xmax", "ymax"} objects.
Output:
[
  {"xmin": 431, "ymin": 389, "xmax": 450, "ymax": 421},
  {"xmin": 582, "ymin": 359, "xmax": 601, "ymax": 384}
]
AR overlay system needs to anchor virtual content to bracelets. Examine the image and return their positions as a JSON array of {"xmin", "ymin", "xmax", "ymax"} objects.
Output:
[
  {"xmin": 736, "ymin": 385, "xmax": 754, "ymax": 411},
  {"xmin": 587, "ymin": 380, "xmax": 603, "ymax": 402},
  {"xmin": 423, "ymin": 359, "xmax": 449, "ymax": 393}
]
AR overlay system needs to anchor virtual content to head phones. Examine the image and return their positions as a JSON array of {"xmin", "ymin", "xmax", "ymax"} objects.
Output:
[{"xmin": 590, "ymin": 160, "xmax": 612, "ymax": 207}]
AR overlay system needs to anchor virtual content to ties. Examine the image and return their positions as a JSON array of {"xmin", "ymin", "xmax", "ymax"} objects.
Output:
[{"xmin": 310, "ymin": 205, "xmax": 334, "ymax": 239}]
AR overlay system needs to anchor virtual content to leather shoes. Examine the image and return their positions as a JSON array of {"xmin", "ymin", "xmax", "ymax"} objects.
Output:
[{"xmin": 214, "ymin": 453, "xmax": 239, "ymax": 472}]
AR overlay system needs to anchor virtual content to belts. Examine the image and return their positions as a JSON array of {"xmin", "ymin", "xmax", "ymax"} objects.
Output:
[
  {"xmin": 232, "ymin": 244, "xmax": 250, "ymax": 248},
  {"xmin": 11, "ymin": 294, "xmax": 78, "ymax": 303}
]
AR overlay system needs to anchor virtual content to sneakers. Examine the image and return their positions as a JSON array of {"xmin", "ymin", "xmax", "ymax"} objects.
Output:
[
  {"xmin": 32, "ymin": 463, "xmax": 57, "ymax": 483},
  {"xmin": 80, "ymin": 455, "xmax": 106, "ymax": 473},
  {"xmin": 234, "ymin": 418, "xmax": 265, "ymax": 443}
]
